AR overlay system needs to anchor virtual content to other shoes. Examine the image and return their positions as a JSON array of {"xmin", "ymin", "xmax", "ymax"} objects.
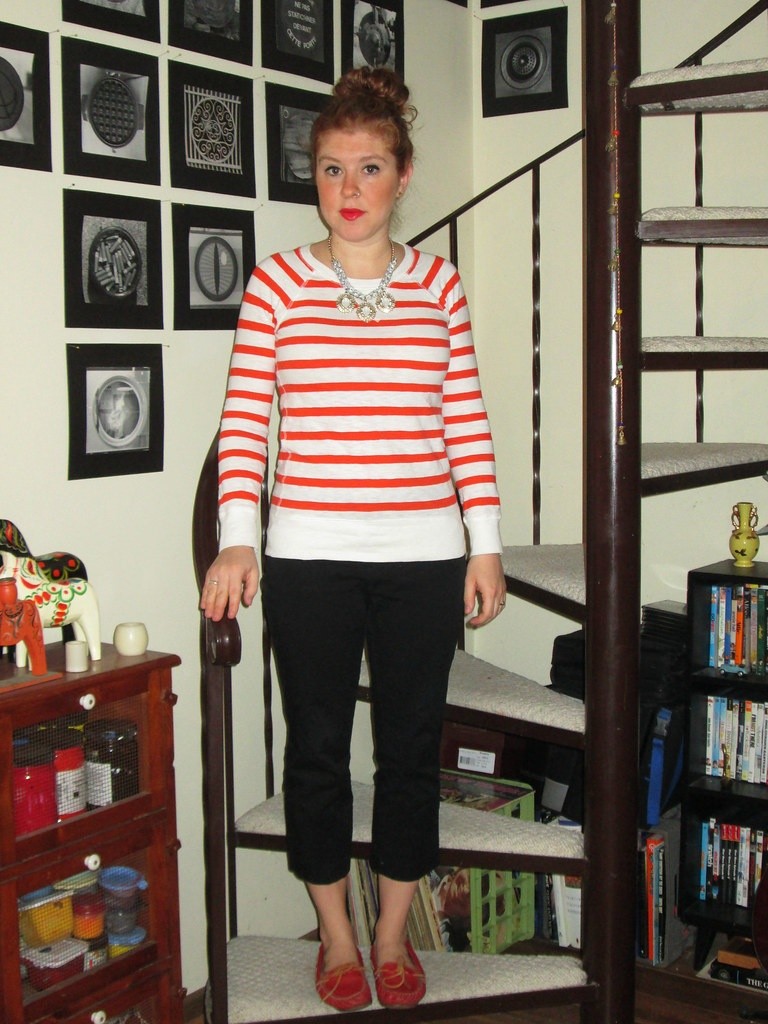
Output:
[
  {"xmin": 370, "ymin": 938, "xmax": 427, "ymax": 1010},
  {"xmin": 315, "ymin": 943, "xmax": 373, "ymax": 1014}
]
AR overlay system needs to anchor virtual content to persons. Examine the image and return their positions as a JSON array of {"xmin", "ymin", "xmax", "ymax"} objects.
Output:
[{"xmin": 200, "ymin": 67, "xmax": 507, "ymax": 1012}]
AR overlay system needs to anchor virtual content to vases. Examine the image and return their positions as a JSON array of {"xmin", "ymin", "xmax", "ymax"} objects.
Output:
[{"xmin": 730, "ymin": 502, "xmax": 758, "ymax": 568}]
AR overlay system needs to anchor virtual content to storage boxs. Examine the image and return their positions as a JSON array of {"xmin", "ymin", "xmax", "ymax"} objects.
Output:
[{"xmin": 441, "ymin": 768, "xmax": 533, "ymax": 956}]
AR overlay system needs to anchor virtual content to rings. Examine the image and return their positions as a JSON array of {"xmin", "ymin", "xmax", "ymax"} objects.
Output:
[
  {"xmin": 500, "ymin": 602, "xmax": 505, "ymax": 605},
  {"xmin": 209, "ymin": 579, "xmax": 218, "ymax": 585}
]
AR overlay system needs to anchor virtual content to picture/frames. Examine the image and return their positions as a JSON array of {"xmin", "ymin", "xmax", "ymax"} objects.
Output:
[{"xmin": 1, "ymin": 0, "xmax": 568, "ymax": 480}]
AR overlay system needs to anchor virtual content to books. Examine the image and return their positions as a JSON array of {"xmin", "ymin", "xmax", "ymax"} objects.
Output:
[
  {"xmin": 636, "ymin": 804, "xmax": 768, "ymax": 991},
  {"xmin": 705, "ymin": 686, "xmax": 768, "ymax": 785},
  {"xmin": 706, "ymin": 583, "xmax": 768, "ymax": 676}
]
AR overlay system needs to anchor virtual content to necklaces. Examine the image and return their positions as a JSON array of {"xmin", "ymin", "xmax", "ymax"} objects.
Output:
[{"xmin": 327, "ymin": 234, "xmax": 398, "ymax": 322}]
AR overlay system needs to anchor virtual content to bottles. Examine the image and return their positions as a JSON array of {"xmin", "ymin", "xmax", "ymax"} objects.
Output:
[
  {"xmin": 728, "ymin": 502, "xmax": 760, "ymax": 568},
  {"xmin": 11, "ymin": 718, "xmax": 140, "ymax": 837}
]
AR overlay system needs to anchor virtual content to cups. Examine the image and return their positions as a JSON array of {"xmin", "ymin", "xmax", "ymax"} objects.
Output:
[{"xmin": 64, "ymin": 640, "xmax": 89, "ymax": 673}]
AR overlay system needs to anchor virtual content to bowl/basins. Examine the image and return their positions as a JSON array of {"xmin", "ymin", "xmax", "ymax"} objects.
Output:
[{"xmin": 16, "ymin": 867, "xmax": 150, "ymax": 991}]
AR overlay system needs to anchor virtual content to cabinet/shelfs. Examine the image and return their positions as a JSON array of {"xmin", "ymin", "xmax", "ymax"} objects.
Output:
[
  {"xmin": 677, "ymin": 559, "xmax": 768, "ymax": 970},
  {"xmin": 0, "ymin": 640, "xmax": 183, "ymax": 1024}
]
